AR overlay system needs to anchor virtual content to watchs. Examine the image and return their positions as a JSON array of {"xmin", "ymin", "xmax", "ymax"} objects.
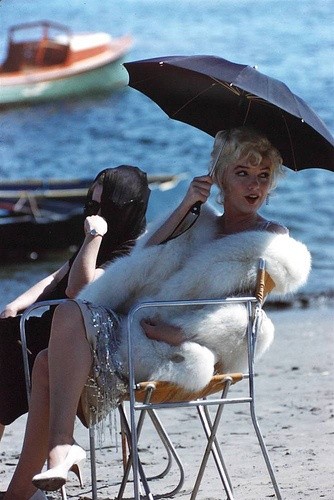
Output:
[{"xmin": 85, "ymin": 228, "xmax": 104, "ymax": 239}]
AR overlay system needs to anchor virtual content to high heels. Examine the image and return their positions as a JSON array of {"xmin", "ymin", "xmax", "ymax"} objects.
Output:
[
  {"xmin": 29, "ymin": 489, "xmax": 48, "ymax": 500},
  {"xmin": 32, "ymin": 441, "xmax": 87, "ymax": 491}
]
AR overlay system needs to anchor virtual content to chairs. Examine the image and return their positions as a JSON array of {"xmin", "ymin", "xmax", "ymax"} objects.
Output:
[{"xmin": 19, "ymin": 256, "xmax": 283, "ymax": 500}]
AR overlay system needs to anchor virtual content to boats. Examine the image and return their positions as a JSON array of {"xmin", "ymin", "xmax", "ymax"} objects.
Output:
[
  {"xmin": 0, "ymin": 170, "xmax": 182, "ymax": 249},
  {"xmin": 0, "ymin": 18, "xmax": 136, "ymax": 107}
]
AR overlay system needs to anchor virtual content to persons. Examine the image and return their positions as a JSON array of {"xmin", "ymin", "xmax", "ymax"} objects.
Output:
[
  {"xmin": 0, "ymin": 164, "xmax": 152, "ymax": 445},
  {"xmin": 2, "ymin": 128, "xmax": 313, "ymax": 500}
]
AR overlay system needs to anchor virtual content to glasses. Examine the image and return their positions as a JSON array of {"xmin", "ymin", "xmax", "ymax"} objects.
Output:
[{"xmin": 84, "ymin": 200, "xmax": 103, "ymax": 217}]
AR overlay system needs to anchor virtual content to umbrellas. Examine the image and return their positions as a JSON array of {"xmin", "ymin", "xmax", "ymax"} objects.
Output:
[{"xmin": 120, "ymin": 53, "xmax": 334, "ymax": 174}]
